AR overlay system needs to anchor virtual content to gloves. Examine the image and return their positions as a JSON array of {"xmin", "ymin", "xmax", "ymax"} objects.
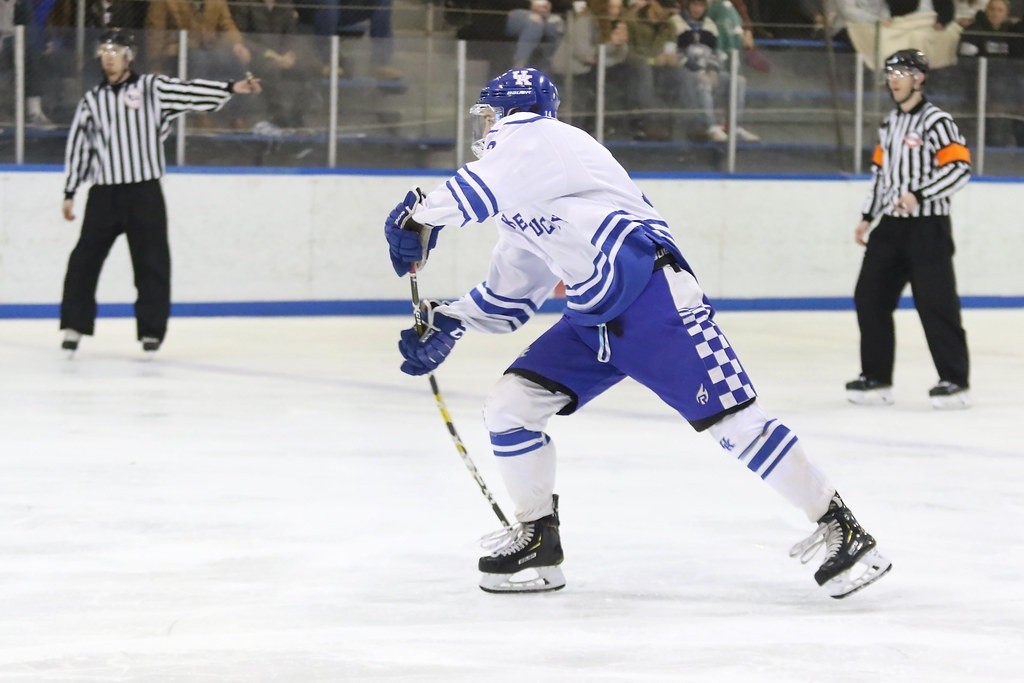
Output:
[
  {"xmin": 398, "ymin": 299, "xmax": 466, "ymax": 376},
  {"xmin": 384, "ymin": 187, "xmax": 444, "ymax": 277}
]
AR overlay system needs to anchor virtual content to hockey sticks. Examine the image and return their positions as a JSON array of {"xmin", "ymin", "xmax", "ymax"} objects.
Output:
[{"xmin": 408, "ymin": 264, "xmax": 511, "ymax": 528}]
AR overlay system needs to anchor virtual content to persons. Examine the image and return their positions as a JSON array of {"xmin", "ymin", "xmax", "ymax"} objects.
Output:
[
  {"xmin": 0, "ymin": 0, "xmax": 409, "ymax": 128},
  {"xmin": 58, "ymin": 27, "xmax": 261, "ymax": 360},
  {"xmin": 845, "ymin": 49, "xmax": 971, "ymax": 410},
  {"xmin": 798, "ymin": 0, "xmax": 1024, "ymax": 105},
  {"xmin": 443, "ymin": 0, "xmax": 760, "ymax": 142},
  {"xmin": 384, "ymin": 67, "xmax": 893, "ymax": 600}
]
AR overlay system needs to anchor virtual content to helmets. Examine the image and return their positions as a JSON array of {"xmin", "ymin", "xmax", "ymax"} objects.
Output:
[
  {"xmin": 885, "ymin": 48, "xmax": 929, "ymax": 76},
  {"xmin": 468, "ymin": 68, "xmax": 560, "ymax": 160},
  {"xmin": 99, "ymin": 26, "xmax": 137, "ymax": 48}
]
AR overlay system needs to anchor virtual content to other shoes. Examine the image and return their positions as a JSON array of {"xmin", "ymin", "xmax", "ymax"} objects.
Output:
[
  {"xmin": 736, "ymin": 126, "xmax": 760, "ymax": 142},
  {"xmin": 708, "ymin": 125, "xmax": 728, "ymax": 142}
]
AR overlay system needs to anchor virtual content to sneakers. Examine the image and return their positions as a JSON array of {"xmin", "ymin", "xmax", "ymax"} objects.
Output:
[
  {"xmin": 788, "ymin": 490, "xmax": 891, "ymax": 598},
  {"xmin": 929, "ymin": 379, "xmax": 970, "ymax": 409},
  {"xmin": 61, "ymin": 328, "xmax": 80, "ymax": 358},
  {"xmin": 141, "ymin": 336, "xmax": 160, "ymax": 358},
  {"xmin": 844, "ymin": 373, "xmax": 894, "ymax": 404},
  {"xmin": 478, "ymin": 494, "xmax": 565, "ymax": 593}
]
